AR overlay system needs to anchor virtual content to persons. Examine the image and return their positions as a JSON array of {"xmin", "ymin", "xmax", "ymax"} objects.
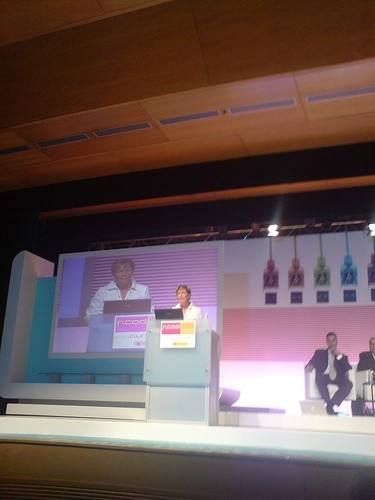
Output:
[
  {"xmin": 170, "ymin": 284, "xmax": 201, "ymax": 320},
  {"xmin": 83, "ymin": 258, "xmax": 155, "ymax": 324},
  {"xmin": 304, "ymin": 332, "xmax": 354, "ymax": 415},
  {"xmin": 356, "ymin": 337, "xmax": 375, "ymax": 385}
]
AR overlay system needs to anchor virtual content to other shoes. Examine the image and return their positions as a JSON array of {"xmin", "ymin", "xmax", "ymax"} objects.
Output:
[{"xmin": 326, "ymin": 405, "xmax": 338, "ymax": 415}]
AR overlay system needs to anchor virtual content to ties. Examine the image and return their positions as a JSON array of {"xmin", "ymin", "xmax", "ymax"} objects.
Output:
[{"xmin": 329, "ymin": 350, "xmax": 337, "ymax": 381}]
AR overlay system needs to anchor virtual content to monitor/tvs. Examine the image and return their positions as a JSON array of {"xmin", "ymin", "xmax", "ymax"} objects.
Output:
[
  {"xmin": 102, "ymin": 298, "xmax": 151, "ymax": 314},
  {"xmin": 154, "ymin": 308, "xmax": 183, "ymax": 320}
]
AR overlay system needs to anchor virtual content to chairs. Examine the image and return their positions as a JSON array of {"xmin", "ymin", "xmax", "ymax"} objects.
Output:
[{"xmin": 306, "ymin": 364, "xmax": 375, "ymax": 416}]
